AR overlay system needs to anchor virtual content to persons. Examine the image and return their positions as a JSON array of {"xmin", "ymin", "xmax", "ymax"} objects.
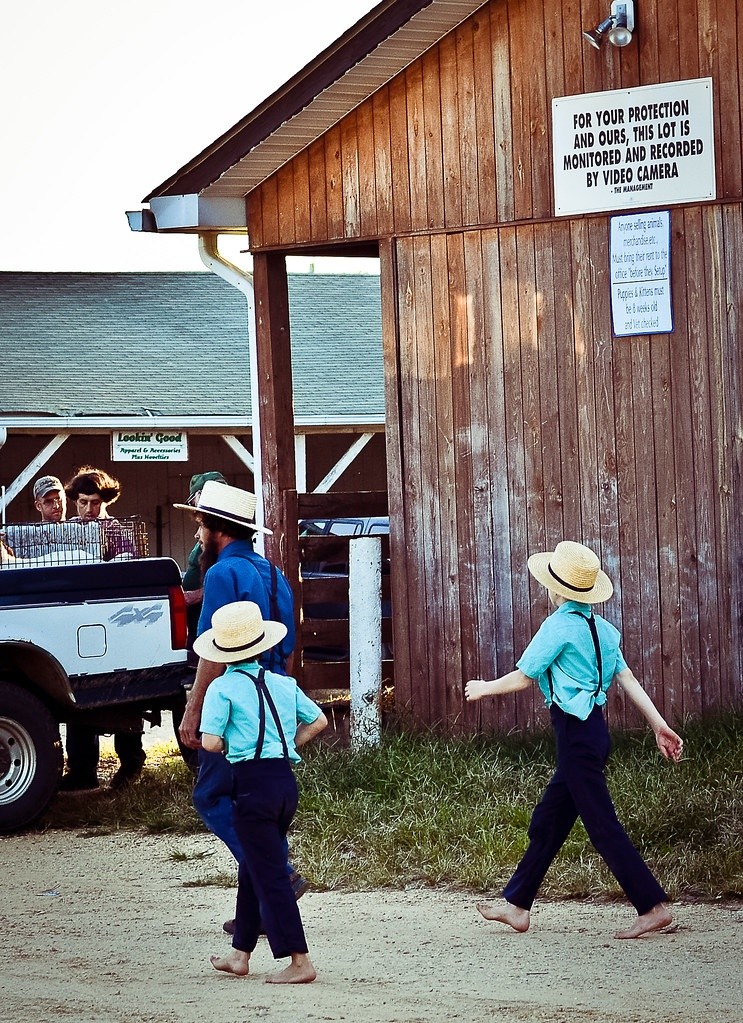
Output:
[
  {"xmin": 465, "ymin": 541, "xmax": 684, "ymax": 939},
  {"xmin": 32, "ymin": 469, "xmax": 145, "ymax": 793},
  {"xmin": 173, "ymin": 479, "xmax": 309, "ymax": 933},
  {"xmin": 193, "ymin": 601, "xmax": 328, "ymax": 984},
  {"xmin": 180, "ymin": 472, "xmax": 229, "ymax": 649}
]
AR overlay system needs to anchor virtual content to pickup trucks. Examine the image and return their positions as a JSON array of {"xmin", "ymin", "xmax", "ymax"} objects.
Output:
[{"xmin": 0, "ymin": 557, "xmax": 191, "ymax": 833}]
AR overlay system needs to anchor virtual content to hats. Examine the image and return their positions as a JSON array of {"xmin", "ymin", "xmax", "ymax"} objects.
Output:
[
  {"xmin": 527, "ymin": 539, "xmax": 614, "ymax": 604},
  {"xmin": 192, "ymin": 601, "xmax": 288, "ymax": 663},
  {"xmin": 33, "ymin": 476, "xmax": 63, "ymax": 499},
  {"xmin": 183, "ymin": 472, "xmax": 225, "ymax": 506},
  {"xmin": 173, "ymin": 483, "xmax": 273, "ymax": 535}
]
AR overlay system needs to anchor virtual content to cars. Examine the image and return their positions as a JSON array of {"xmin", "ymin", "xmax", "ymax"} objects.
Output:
[{"xmin": 297, "ymin": 517, "xmax": 390, "ymax": 661}]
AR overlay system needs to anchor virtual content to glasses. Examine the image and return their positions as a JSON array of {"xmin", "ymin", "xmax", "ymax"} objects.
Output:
[{"xmin": 188, "ymin": 490, "xmax": 200, "ymax": 508}]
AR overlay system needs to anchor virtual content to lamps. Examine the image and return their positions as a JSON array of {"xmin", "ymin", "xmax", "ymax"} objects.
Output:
[{"xmin": 582, "ymin": 0, "xmax": 635, "ymax": 49}]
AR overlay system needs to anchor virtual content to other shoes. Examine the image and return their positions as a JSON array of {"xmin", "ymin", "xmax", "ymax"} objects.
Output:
[
  {"xmin": 289, "ymin": 873, "xmax": 308, "ymax": 899},
  {"xmin": 62, "ymin": 771, "xmax": 101, "ymax": 792},
  {"xmin": 110, "ymin": 753, "xmax": 146, "ymax": 790},
  {"xmin": 222, "ymin": 918, "xmax": 268, "ymax": 936}
]
